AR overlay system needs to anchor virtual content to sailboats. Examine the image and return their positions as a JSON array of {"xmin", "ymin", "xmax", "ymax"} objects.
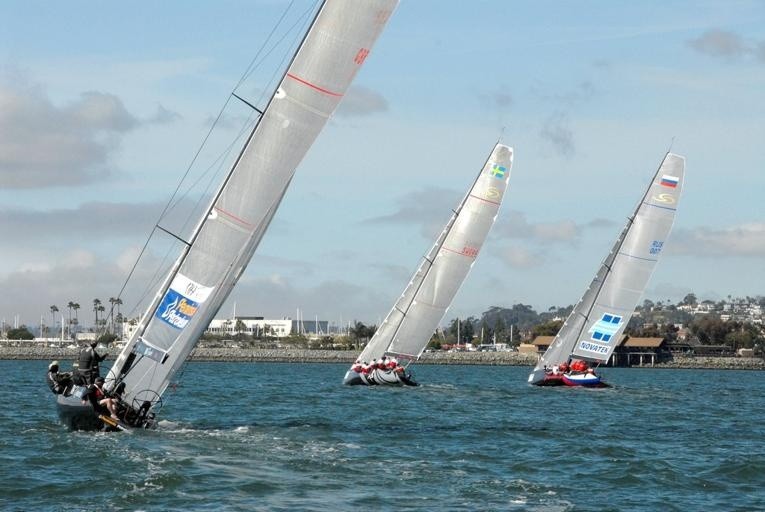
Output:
[
  {"xmin": 57, "ymin": 0, "xmax": 400, "ymax": 432},
  {"xmin": 528, "ymin": 153, "xmax": 685, "ymax": 386},
  {"xmin": 342, "ymin": 143, "xmax": 514, "ymax": 386}
]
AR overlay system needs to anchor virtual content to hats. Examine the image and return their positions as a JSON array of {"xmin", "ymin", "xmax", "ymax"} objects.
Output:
[
  {"xmin": 95, "ymin": 377, "xmax": 105, "ymax": 383},
  {"xmin": 91, "ymin": 341, "xmax": 97, "ymax": 344},
  {"xmin": 52, "ymin": 360, "xmax": 59, "ymax": 366}
]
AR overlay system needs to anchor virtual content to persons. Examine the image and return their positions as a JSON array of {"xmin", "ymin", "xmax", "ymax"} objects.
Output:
[
  {"xmin": 46, "ymin": 340, "xmax": 120, "ymax": 421},
  {"xmin": 543, "ymin": 359, "xmax": 589, "ymax": 374},
  {"xmin": 351, "ymin": 356, "xmax": 405, "ymax": 375}
]
